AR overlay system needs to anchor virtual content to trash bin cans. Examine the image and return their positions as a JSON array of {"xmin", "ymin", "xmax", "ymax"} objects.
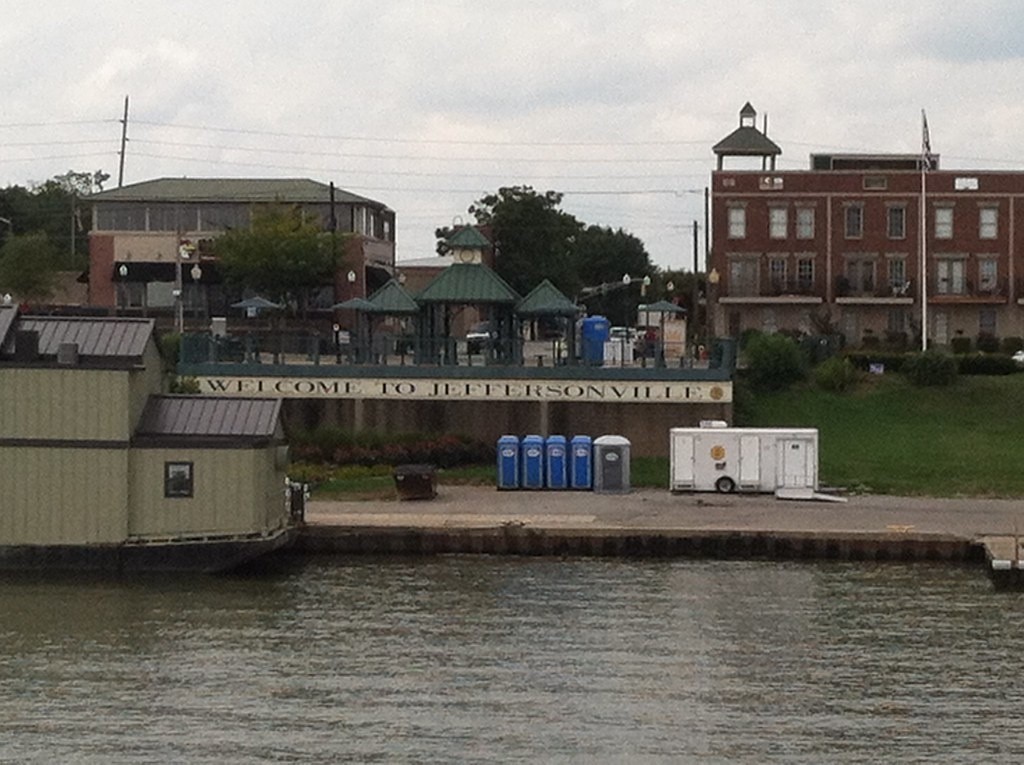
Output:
[
  {"xmin": 583, "ymin": 315, "xmax": 611, "ymax": 365},
  {"xmin": 496, "ymin": 435, "xmax": 631, "ymax": 494}
]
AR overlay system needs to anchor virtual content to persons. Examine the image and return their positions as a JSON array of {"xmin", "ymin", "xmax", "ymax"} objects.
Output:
[
  {"xmin": 862, "ymin": 328, "xmax": 880, "ymax": 350},
  {"xmin": 952, "ymin": 329, "xmax": 971, "ymax": 358},
  {"xmin": 338, "ymin": 317, "xmax": 415, "ymax": 365}
]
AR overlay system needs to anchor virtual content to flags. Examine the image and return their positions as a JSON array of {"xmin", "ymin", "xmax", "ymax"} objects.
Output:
[{"xmin": 922, "ymin": 115, "xmax": 932, "ymax": 174}]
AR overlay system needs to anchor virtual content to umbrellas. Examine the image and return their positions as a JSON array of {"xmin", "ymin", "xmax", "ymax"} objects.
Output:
[
  {"xmin": 332, "ymin": 297, "xmax": 378, "ymax": 311},
  {"xmin": 230, "ymin": 296, "xmax": 281, "ymax": 308}
]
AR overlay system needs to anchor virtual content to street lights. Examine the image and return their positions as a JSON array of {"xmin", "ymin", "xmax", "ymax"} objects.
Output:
[
  {"xmin": 347, "ymin": 270, "xmax": 357, "ymax": 364},
  {"xmin": 119, "ymin": 264, "xmax": 128, "ymax": 316},
  {"xmin": 622, "ymin": 272, "xmax": 631, "ymax": 344},
  {"xmin": 190, "ymin": 264, "xmax": 203, "ymax": 363}
]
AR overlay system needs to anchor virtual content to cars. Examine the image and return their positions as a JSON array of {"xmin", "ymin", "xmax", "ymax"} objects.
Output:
[{"xmin": 466, "ymin": 320, "xmax": 508, "ymax": 355}]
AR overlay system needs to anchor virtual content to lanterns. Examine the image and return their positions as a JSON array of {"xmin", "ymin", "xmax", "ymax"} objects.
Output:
[
  {"xmin": 191, "ymin": 265, "xmax": 201, "ymax": 279},
  {"xmin": 119, "ymin": 265, "xmax": 128, "ymax": 276},
  {"xmin": 348, "ymin": 270, "xmax": 355, "ymax": 283}
]
